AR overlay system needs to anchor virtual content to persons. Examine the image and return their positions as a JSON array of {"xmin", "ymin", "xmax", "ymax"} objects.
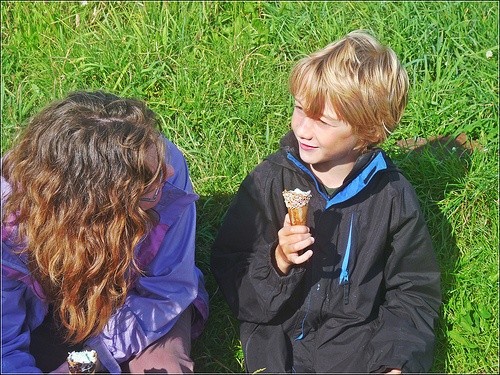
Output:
[
  {"xmin": 0, "ymin": 89, "xmax": 209, "ymax": 375},
  {"xmin": 209, "ymin": 28, "xmax": 442, "ymax": 375}
]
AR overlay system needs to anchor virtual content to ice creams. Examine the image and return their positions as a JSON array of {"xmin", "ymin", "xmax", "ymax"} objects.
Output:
[
  {"xmin": 67, "ymin": 350, "xmax": 98, "ymax": 374},
  {"xmin": 282, "ymin": 187, "xmax": 312, "ymax": 226}
]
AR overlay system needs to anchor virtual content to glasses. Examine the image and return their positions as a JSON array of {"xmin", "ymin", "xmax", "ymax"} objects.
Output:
[{"xmin": 139, "ymin": 155, "xmax": 163, "ymax": 201}]
